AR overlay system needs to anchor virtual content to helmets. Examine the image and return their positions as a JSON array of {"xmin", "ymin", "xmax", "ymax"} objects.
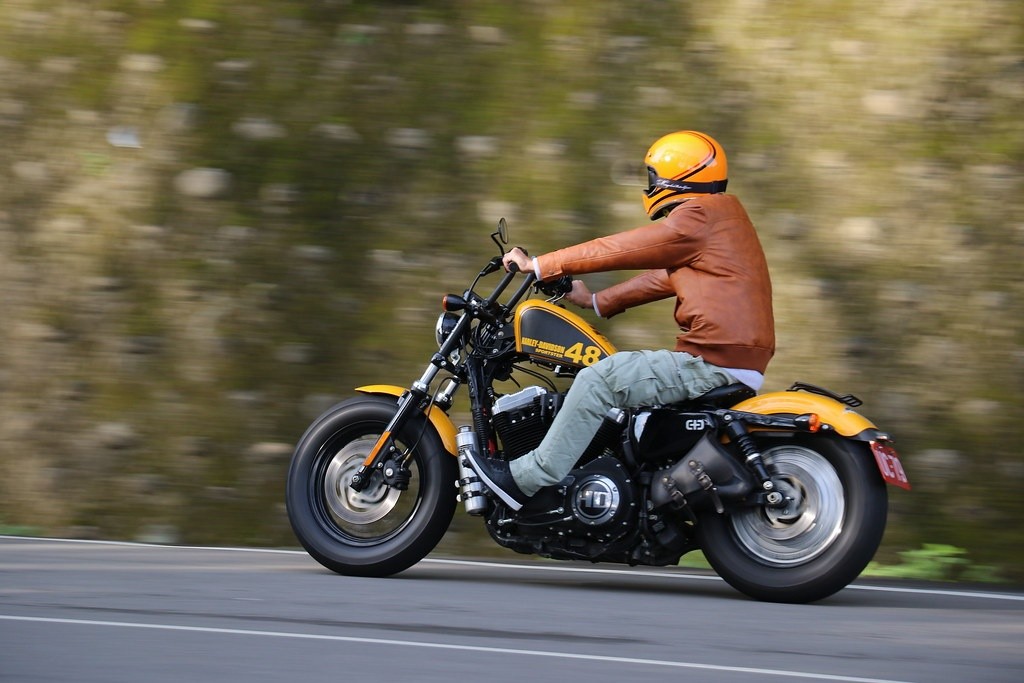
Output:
[{"xmin": 642, "ymin": 130, "xmax": 728, "ymax": 221}]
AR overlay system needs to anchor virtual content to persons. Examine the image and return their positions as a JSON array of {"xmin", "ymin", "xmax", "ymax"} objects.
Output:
[{"xmin": 464, "ymin": 130, "xmax": 775, "ymax": 511}]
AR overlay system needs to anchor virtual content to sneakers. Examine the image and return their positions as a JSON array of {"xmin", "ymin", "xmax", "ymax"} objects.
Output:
[{"xmin": 465, "ymin": 449, "xmax": 530, "ymax": 510}]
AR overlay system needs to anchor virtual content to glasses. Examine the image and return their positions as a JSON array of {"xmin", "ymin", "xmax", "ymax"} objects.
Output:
[{"xmin": 647, "ymin": 166, "xmax": 659, "ymax": 193}]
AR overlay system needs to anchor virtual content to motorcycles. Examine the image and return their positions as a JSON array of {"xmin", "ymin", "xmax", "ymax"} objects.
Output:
[{"xmin": 283, "ymin": 219, "xmax": 912, "ymax": 606}]
